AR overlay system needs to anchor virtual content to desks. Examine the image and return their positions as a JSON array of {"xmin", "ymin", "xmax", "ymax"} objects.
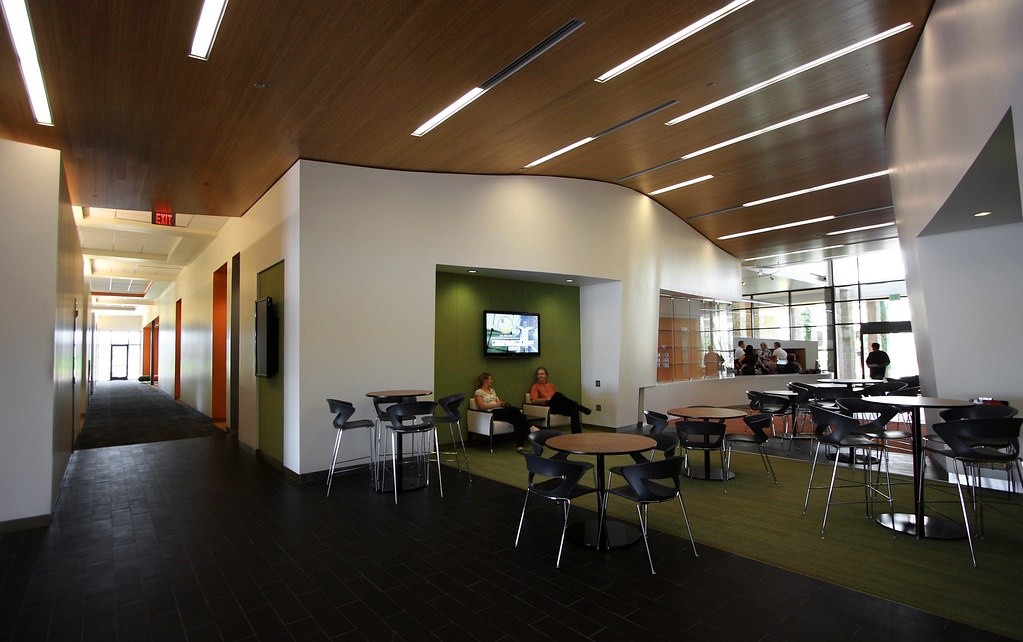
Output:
[
  {"xmin": 669, "ymin": 408, "xmax": 747, "ymax": 480},
  {"xmin": 366, "ymin": 390, "xmax": 432, "ymax": 492},
  {"xmin": 545, "ymin": 433, "xmax": 656, "ymax": 550},
  {"xmin": 816, "ymin": 378, "xmax": 884, "ymax": 463},
  {"xmin": 764, "ymin": 390, "xmax": 814, "ymax": 439},
  {"xmin": 859, "ymin": 394, "xmax": 978, "ymax": 538}
]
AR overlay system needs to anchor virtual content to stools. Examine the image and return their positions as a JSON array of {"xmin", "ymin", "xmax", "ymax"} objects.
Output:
[
  {"xmin": 513, "ymin": 375, "xmax": 920, "ymax": 584},
  {"xmin": 801, "ymin": 398, "xmax": 1023, "ymax": 569},
  {"xmin": 325, "ymin": 394, "xmax": 470, "ymax": 504}
]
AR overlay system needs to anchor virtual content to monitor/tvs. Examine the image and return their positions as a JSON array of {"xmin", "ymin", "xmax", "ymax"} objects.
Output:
[
  {"xmin": 483, "ymin": 310, "xmax": 541, "ymax": 358},
  {"xmin": 255, "ymin": 296, "xmax": 279, "ymax": 379}
]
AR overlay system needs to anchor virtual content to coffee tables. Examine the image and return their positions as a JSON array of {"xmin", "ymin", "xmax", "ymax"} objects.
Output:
[{"xmin": 526, "ymin": 415, "xmax": 543, "ymax": 421}]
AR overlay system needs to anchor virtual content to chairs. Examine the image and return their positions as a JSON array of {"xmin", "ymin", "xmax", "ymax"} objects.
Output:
[
  {"xmin": 466, "ymin": 398, "xmax": 523, "ymax": 452},
  {"xmin": 525, "ymin": 393, "xmax": 574, "ymax": 431}
]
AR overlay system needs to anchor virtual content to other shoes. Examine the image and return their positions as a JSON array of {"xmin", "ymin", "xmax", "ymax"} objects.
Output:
[
  {"xmin": 530, "ymin": 426, "xmax": 540, "ymax": 433},
  {"xmin": 517, "ymin": 449, "xmax": 528, "ymax": 454},
  {"xmin": 583, "ymin": 408, "xmax": 591, "ymax": 415}
]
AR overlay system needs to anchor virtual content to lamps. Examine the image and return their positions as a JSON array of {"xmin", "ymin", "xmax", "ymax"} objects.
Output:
[{"xmin": 757, "ymin": 264, "xmax": 764, "ymax": 277}]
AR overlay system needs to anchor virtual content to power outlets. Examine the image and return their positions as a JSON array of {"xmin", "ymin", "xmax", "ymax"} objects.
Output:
[{"xmin": 595, "ymin": 405, "xmax": 601, "ymax": 412}]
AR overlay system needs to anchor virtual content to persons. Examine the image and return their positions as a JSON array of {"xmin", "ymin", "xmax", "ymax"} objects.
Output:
[
  {"xmin": 530, "ymin": 367, "xmax": 591, "ymax": 434},
  {"xmin": 866, "ymin": 343, "xmax": 890, "ymax": 380},
  {"xmin": 734, "ymin": 341, "xmax": 802, "ymax": 373},
  {"xmin": 702, "ymin": 346, "xmax": 720, "ymax": 374},
  {"xmin": 514, "ymin": 321, "xmax": 536, "ymax": 340},
  {"xmin": 474, "ymin": 372, "xmax": 541, "ymax": 454}
]
